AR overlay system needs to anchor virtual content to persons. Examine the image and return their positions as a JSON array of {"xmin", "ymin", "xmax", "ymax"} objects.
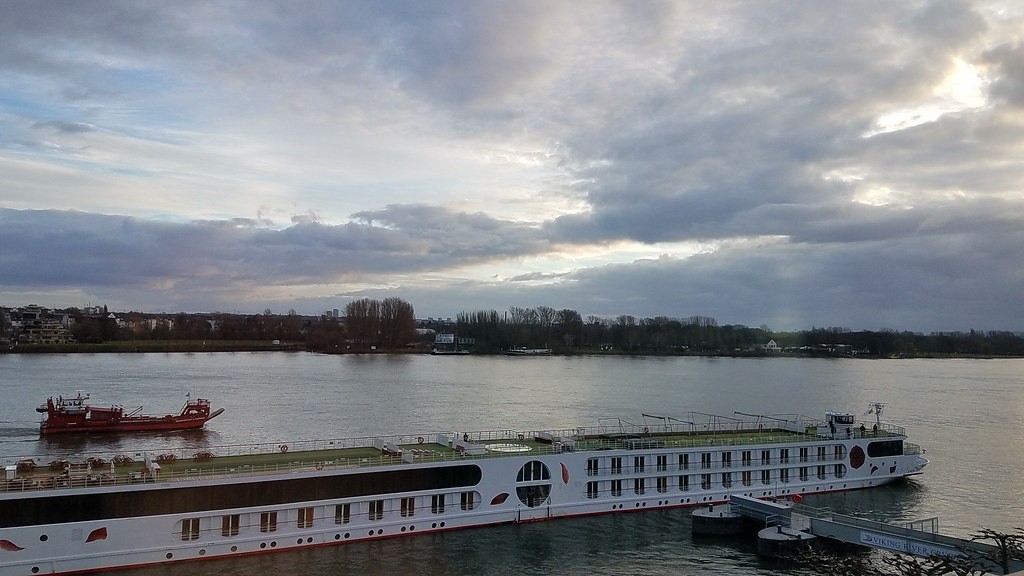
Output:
[
  {"xmin": 860, "ymin": 425, "xmax": 866, "ymax": 436},
  {"xmin": 831, "ymin": 425, "xmax": 837, "ymax": 439},
  {"xmin": 873, "ymin": 424, "xmax": 877, "ymax": 436},
  {"xmin": 846, "ymin": 426, "xmax": 852, "ymax": 438}
]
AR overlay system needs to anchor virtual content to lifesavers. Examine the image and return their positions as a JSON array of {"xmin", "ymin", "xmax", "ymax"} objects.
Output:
[
  {"xmin": 519, "ymin": 434, "xmax": 524, "ymax": 439},
  {"xmin": 316, "ymin": 462, "xmax": 323, "ymax": 470},
  {"xmin": 280, "ymin": 445, "xmax": 288, "ymax": 452},
  {"xmin": 418, "ymin": 437, "xmax": 424, "ymax": 443}
]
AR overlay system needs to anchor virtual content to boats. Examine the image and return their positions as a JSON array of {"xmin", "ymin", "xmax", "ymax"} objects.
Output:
[
  {"xmin": 36, "ymin": 388, "xmax": 225, "ymax": 434},
  {"xmin": 1, "ymin": 396, "xmax": 930, "ymax": 576}
]
